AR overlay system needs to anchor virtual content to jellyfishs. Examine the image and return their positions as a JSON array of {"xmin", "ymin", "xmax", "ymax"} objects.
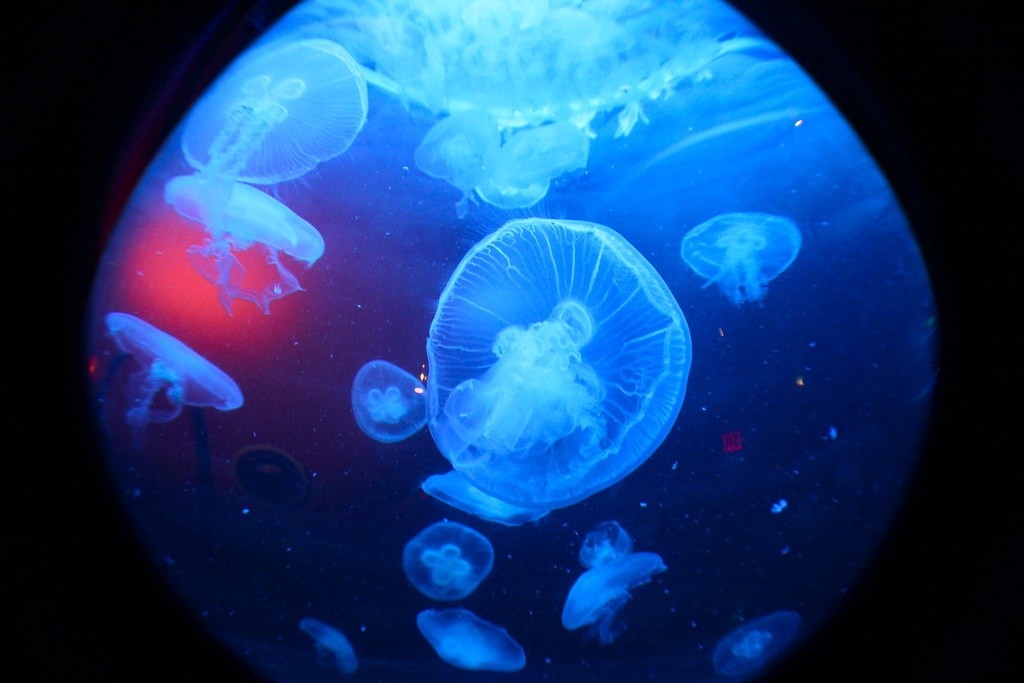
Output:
[{"xmin": 91, "ymin": 41, "xmax": 929, "ymax": 671}]
